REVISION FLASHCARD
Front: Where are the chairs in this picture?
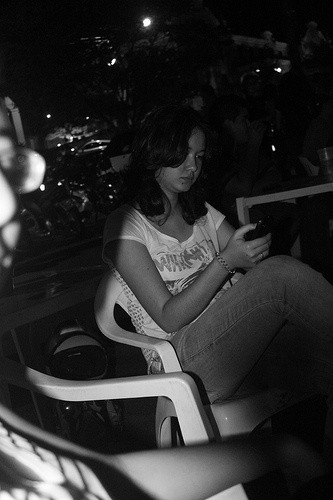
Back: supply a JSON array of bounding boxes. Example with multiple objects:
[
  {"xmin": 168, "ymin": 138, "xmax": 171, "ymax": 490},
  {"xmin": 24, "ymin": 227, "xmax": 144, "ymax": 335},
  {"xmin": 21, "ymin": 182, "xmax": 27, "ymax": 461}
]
[
  {"xmin": 1, "ymin": 358, "xmax": 248, "ymax": 500},
  {"xmin": 94, "ymin": 272, "xmax": 314, "ymax": 450}
]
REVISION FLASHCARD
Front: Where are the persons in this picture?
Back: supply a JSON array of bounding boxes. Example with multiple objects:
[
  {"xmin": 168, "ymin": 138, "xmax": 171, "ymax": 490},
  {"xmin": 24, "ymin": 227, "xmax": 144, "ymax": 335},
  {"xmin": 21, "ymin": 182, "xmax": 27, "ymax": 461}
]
[
  {"xmin": 0, "ymin": 95, "xmax": 331, "ymax": 500},
  {"xmin": 101, "ymin": 105, "xmax": 333, "ymax": 472},
  {"xmin": 75, "ymin": 1, "xmax": 333, "ymax": 284}
]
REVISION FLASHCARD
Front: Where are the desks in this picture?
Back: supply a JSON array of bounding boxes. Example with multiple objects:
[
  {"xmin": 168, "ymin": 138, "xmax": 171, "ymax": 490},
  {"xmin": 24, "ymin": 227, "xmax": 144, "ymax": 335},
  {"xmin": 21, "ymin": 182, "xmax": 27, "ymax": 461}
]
[
  {"xmin": 235, "ymin": 176, "xmax": 333, "ymax": 228},
  {"xmin": 0, "ymin": 275, "xmax": 102, "ymax": 433}
]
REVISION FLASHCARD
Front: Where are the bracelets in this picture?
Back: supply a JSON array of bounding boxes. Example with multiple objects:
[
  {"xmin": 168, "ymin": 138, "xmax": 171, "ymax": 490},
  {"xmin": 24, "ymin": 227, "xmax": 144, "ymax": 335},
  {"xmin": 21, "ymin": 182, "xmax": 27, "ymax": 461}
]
[{"xmin": 214, "ymin": 252, "xmax": 236, "ymax": 274}]
